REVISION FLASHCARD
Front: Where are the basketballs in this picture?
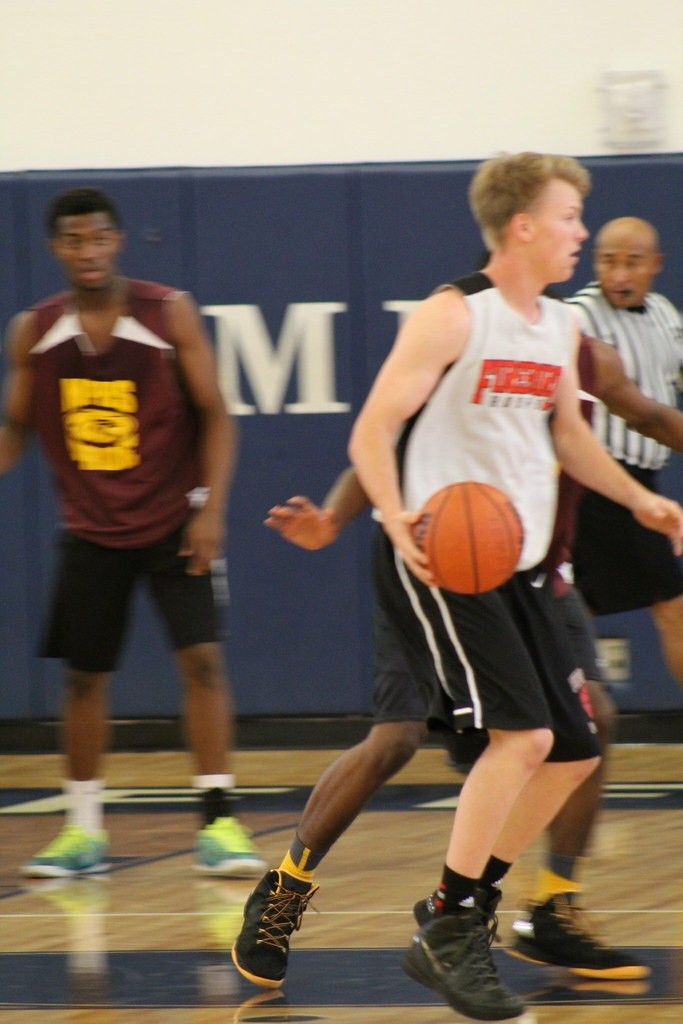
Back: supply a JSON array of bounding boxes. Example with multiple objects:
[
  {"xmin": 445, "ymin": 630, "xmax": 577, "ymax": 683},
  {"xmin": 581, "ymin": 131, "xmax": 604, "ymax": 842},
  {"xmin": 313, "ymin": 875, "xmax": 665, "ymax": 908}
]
[{"xmin": 410, "ymin": 481, "xmax": 525, "ymax": 595}]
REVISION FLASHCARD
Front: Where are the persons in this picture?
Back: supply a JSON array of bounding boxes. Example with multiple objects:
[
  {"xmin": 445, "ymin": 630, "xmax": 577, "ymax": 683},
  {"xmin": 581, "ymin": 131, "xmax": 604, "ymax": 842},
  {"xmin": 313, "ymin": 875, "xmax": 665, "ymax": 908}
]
[
  {"xmin": 232, "ymin": 250, "xmax": 683, "ymax": 989},
  {"xmin": 349, "ymin": 149, "xmax": 683, "ymax": 1021},
  {"xmin": 0, "ymin": 189, "xmax": 269, "ymax": 880},
  {"xmin": 562, "ymin": 217, "xmax": 683, "ymax": 679}
]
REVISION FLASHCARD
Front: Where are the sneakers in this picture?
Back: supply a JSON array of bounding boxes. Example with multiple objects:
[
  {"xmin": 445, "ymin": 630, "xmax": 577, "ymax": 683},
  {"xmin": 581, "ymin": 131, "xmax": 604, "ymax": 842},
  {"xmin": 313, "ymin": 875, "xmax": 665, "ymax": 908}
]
[
  {"xmin": 413, "ymin": 893, "xmax": 440, "ymax": 926},
  {"xmin": 15, "ymin": 822, "xmax": 113, "ymax": 879},
  {"xmin": 507, "ymin": 867, "xmax": 653, "ymax": 980},
  {"xmin": 399, "ymin": 909, "xmax": 526, "ymax": 1020},
  {"xmin": 190, "ymin": 816, "xmax": 269, "ymax": 881},
  {"xmin": 231, "ymin": 868, "xmax": 321, "ymax": 988}
]
